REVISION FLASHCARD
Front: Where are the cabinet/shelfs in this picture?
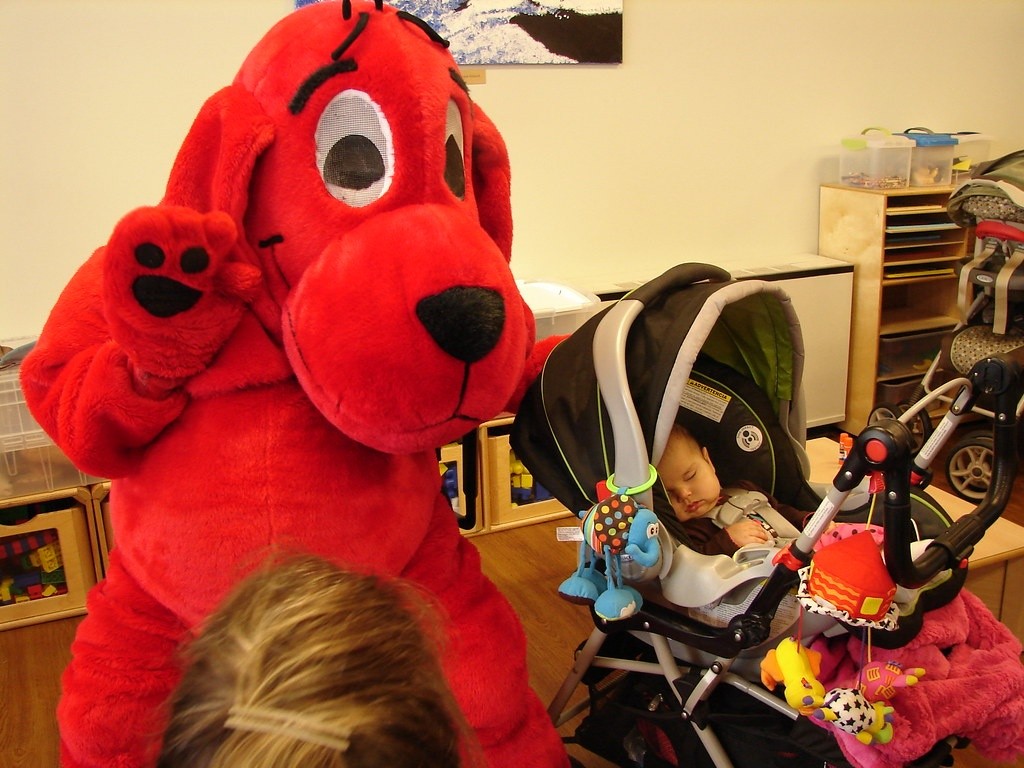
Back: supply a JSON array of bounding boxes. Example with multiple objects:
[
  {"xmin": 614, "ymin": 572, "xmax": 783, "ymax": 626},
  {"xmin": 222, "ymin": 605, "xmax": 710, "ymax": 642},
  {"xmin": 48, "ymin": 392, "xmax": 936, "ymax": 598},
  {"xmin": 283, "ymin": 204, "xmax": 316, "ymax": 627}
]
[{"xmin": 819, "ymin": 175, "xmax": 977, "ymax": 437}]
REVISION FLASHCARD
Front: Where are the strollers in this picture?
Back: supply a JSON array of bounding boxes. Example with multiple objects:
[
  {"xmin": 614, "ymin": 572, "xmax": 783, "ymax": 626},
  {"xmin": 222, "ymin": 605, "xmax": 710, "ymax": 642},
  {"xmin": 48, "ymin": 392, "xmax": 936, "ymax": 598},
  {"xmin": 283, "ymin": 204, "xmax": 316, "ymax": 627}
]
[
  {"xmin": 863, "ymin": 149, "xmax": 1024, "ymax": 505},
  {"xmin": 506, "ymin": 261, "xmax": 1024, "ymax": 768}
]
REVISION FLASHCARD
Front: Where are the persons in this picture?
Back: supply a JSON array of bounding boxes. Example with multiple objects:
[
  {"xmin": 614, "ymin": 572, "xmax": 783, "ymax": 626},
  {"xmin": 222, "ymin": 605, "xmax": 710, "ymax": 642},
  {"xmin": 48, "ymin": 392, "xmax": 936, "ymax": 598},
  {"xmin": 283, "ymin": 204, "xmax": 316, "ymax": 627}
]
[
  {"xmin": 656, "ymin": 423, "xmax": 836, "ymax": 559},
  {"xmin": 150, "ymin": 551, "xmax": 484, "ymax": 768}
]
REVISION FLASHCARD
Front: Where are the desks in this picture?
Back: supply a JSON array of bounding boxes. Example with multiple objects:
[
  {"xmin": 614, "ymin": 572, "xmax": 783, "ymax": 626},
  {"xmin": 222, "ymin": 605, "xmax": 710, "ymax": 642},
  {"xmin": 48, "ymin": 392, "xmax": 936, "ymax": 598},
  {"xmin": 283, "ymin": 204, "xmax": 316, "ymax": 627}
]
[{"xmin": 803, "ymin": 437, "xmax": 1024, "ymax": 642}]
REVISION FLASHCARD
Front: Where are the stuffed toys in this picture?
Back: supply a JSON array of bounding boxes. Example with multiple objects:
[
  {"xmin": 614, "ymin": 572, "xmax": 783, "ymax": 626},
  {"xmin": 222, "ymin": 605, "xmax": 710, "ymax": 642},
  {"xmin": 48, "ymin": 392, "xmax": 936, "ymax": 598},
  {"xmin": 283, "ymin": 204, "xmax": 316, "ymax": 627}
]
[
  {"xmin": 813, "ymin": 626, "xmax": 894, "ymax": 747},
  {"xmin": 856, "ymin": 626, "xmax": 925, "ymax": 701},
  {"xmin": 759, "ymin": 603, "xmax": 838, "ymax": 721},
  {"xmin": 558, "ymin": 486, "xmax": 661, "ymax": 622},
  {"xmin": 18, "ymin": 1, "xmax": 578, "ymax": 768}
]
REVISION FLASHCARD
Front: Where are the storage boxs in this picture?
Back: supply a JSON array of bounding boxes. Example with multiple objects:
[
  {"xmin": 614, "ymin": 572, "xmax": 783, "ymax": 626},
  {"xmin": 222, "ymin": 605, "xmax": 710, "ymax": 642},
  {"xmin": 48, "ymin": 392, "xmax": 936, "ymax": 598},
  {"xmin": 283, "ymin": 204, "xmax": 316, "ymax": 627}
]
[
  {"xmin": 0, "ymin": 360, "xmax": 111, "ymax": 500},
  {"xmin": 892, "ymin": 126, "xmax": 958, "ymax": 187},
  {"xmin": 840, "ymin": 127, "xmax": 916, "ymax": 189},
  {"xmin": 487, "ymin": 432, "xmax": 575, "ymax": 526},
  {"xmin": 951, "ymin": 133, "xmax": 993, "ymax": 186},
  {"xmin": 438, "ymin": 441, "xmax": 482, "ymax": 535},
  {"xmin": 0, "ymin": 506, "xmax": 95, "ymax": 624}
]
